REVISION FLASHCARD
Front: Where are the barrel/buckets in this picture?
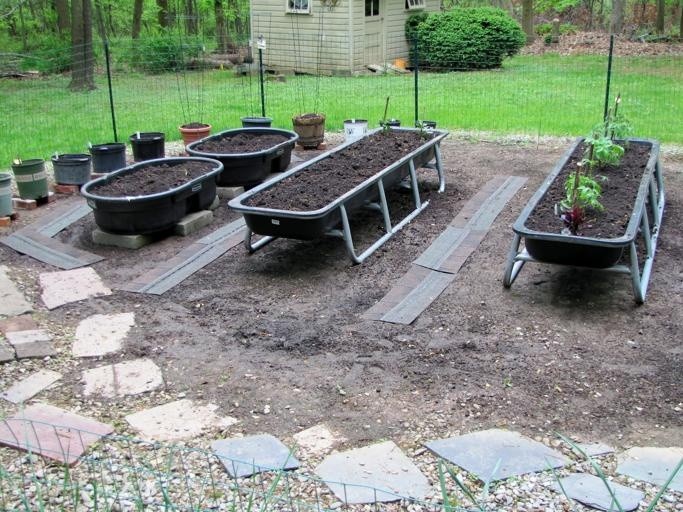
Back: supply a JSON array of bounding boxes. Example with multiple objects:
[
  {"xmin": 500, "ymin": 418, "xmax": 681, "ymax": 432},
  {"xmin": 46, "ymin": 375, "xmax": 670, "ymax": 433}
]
[
  {"xmin": 292, "ymin": 111, "xmax": 328, "ymax": 149},
  {"xmin": 0, "ymin": 174, "xmax": 12, "ymax": 218},
  {"xmin": 13, "ymin": 158, "xmax": 49, "ymax": 199},
  {"xmin": 243, "ymin": 115, "xmax": 270, "ymax": 128},
  {"xmin": 423, "ymin": 121, "xmax": 436, "ymax": 130},
  {"xmin": 129, "ymin": 128, "xmax": 167, "ymax": 159},
  {"xmin": 90, "ymin": 141, "xmax": 127, "ymax": 173},
  {"xmin": 51, "ymin": 153, "xmax": 91, "ymax": 187},
  {"xmin": 380, "ymin": 118, "xmax": 402, "ymax": 126},
  {"xmin": 343, "ymin": 119, "xmax": 367, "ymax": 141}
]
